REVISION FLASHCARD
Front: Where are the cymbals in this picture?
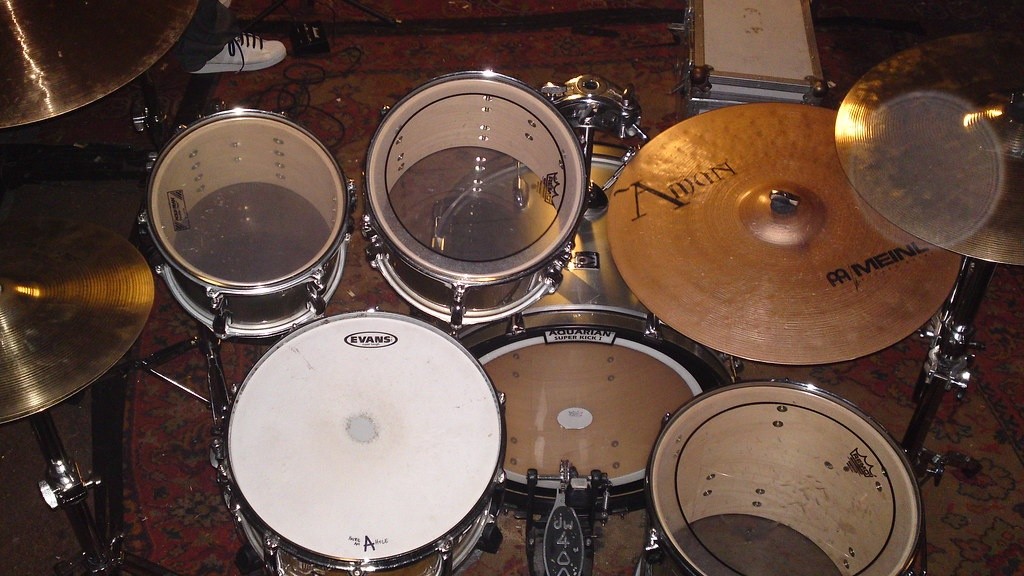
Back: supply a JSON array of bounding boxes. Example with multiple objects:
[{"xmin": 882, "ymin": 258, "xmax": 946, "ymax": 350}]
[
  {"xmin": 606, "ymin": 100, "xmax": 963, "ymax": 366},
  {"xmin": 834, "ymin": 30, "xmax": 1024, "ymax": 266},
  {"xmin": 0, "ymin": 212, "xmax": 155, "ymax": 425},
  {"xmin": 0, "ymin": 1, "xmax": 202, "ymax": 128}
]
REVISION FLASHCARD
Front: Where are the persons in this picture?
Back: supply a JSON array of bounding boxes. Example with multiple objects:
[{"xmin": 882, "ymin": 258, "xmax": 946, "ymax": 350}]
[{"xmin": 171, "ymin": 0, "xmax": 286, "ymax": 75}]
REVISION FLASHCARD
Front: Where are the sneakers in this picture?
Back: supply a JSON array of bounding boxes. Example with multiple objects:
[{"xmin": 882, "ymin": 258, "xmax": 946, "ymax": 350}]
[{"xmin": 190, "ymin": 32, "xmax": 288, "ymax": 73}]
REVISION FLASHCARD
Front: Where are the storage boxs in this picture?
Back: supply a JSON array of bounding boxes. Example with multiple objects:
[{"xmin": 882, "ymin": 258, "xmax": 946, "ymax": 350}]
[{"xmin": 666, "ymin": 0, "xmax": 830, "ymax": 123}]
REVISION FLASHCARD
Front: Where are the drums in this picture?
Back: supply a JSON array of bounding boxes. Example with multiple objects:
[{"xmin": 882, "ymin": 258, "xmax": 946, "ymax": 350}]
[
  {"xmin": 412, "ymin": 140, "xmax": 739, "ymax": 520},
  {"xmin": 217, "ymin": 308, "xmax": 507, "ymax": 576},
  {"xmin": 644, "ymin": 380, "xmax": 926, "ymax": 576},
  {"xmin": 368, "ymin": 68, "xmax": 594, "ymax": 325},
  {"xmin": 137, "ymin": 108, "xmax": 358, "ymax": 340}
]
